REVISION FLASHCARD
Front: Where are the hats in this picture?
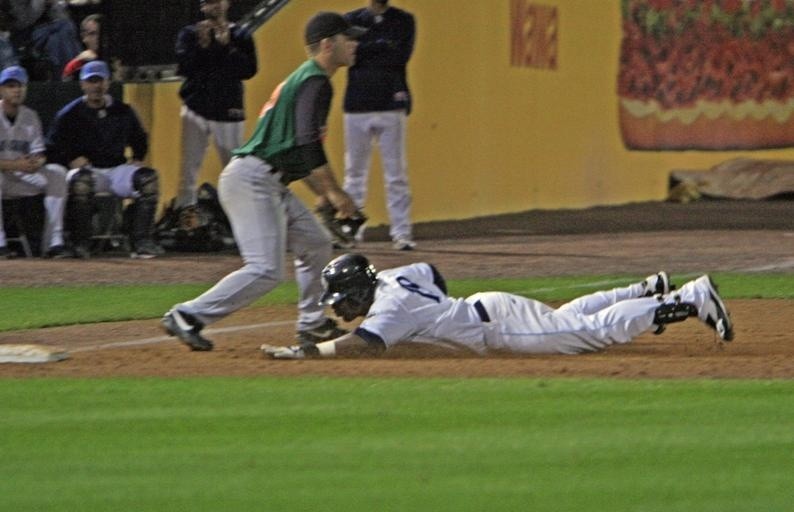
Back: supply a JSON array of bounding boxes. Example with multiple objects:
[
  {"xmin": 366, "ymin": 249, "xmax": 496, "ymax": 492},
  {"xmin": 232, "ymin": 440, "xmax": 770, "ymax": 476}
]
[
  {"xmin": 81, "ymin": 62, "xmax": 109, "ymax": 81},
  {"xmin": 0, "ymin": 65, "xmax": 28, "ymax": 84},
  {"xmin": 304, "ymin": 11, "xmax": 369, "ymax": 45}
]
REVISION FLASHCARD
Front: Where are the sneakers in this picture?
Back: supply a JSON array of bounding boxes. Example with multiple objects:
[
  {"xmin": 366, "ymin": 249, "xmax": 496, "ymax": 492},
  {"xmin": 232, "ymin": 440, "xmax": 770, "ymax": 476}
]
[
  {"xmin": 129, "ymin": 246, "xmax": 158, "ymax": 259},
  {"xmin": 393, "ymin": 234, "xmax": 416, "ymax": 251},
  {"xmin": 50, "ymin": 245, "xmax": 75, "ymax": 259},
  {"xmin": 161, "ymin": 310, "xmax": 213, "ymax": 352},
  {"xmin": 693, "ymin": 274, "xmax": 736, "ymax": 342},
  {"xmin": 295, "ymin": 318, "xmax": 349, "ymax": 341},
  {"xmin": 642, "ymin": 268, "xmax": 671, "ymax": 294}
]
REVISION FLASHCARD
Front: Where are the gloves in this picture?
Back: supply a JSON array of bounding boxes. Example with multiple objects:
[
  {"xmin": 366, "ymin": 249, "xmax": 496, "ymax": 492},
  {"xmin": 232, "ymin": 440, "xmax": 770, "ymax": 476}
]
[{"xmin": 261, "ymin": 342, "xmax": 335, "ymax": 360}]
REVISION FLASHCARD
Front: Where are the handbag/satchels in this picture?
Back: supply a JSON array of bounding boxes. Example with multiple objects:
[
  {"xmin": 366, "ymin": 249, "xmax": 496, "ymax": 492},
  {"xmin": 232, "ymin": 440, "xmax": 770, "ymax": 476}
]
[{"xmin": 174, "ymin": 204, "xmax": 224, "ymax": 252}]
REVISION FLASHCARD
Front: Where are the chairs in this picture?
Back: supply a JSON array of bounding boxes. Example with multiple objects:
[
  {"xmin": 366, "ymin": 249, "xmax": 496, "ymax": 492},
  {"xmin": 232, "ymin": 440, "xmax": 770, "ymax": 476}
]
[
  {"xmin": 47, "ymin": 189, "xmax": 139, "ymax": 257},
  {"xmin": 0, "ymin": 194, "xmax": 32, "ymax": 259}
]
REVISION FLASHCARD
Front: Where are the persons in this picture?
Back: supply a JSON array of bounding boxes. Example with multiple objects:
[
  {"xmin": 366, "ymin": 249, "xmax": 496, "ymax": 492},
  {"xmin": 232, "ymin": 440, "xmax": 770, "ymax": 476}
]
[
  {"xmin": 259, "ymin": 250, "xmax": 735, "ymax": 364},
  {"xmin": 341, "ymin": 0, "xmax": 417, "ymax": 251},
  {"xmin": 159, "ymin": 8, "xmax": 368, "ymax": 354},
  {"xmin": 1, "ymin": 1, "xmax": 257, "ymax": 258}
]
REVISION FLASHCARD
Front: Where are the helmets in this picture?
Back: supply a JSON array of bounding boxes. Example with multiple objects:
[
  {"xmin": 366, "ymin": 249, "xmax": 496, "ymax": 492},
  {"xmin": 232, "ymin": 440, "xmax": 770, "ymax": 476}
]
[{"xmin": 318, "ymin": 254, "xmax": 377, "ymax": 305}]
